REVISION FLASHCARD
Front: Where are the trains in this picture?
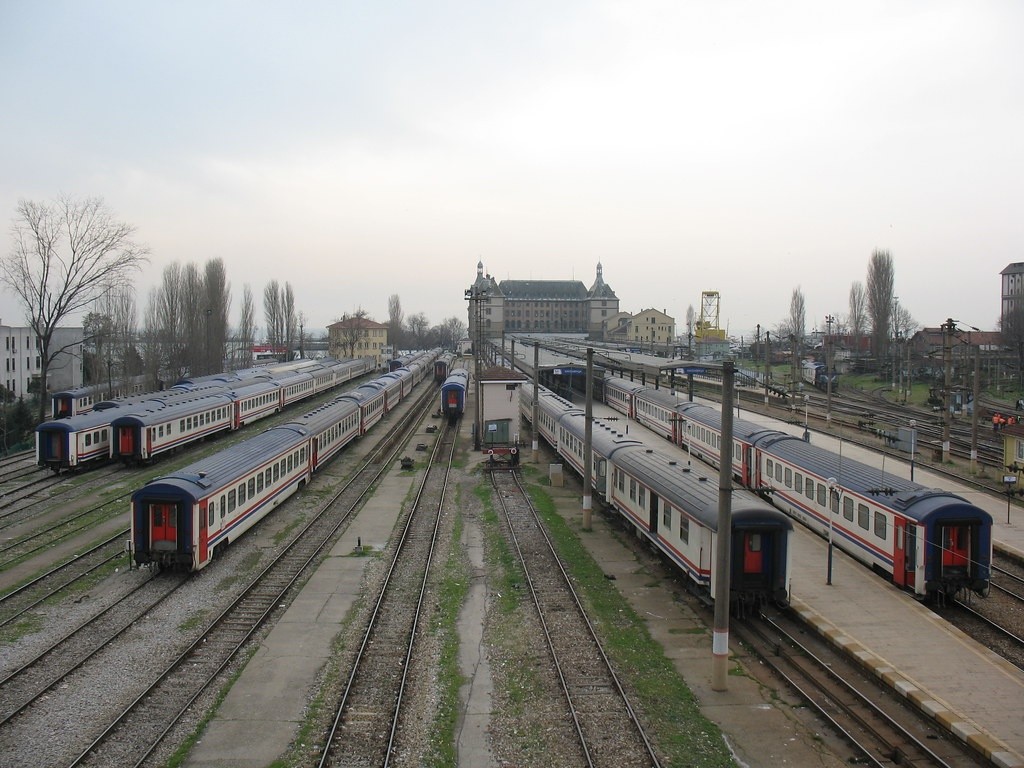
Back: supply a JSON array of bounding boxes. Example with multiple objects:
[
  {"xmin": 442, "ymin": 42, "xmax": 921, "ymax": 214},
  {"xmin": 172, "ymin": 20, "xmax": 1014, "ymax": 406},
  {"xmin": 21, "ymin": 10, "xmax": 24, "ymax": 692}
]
[
  {"xmin": 803, "ymin": 362, "xmax": 838, "ymax": 393},
  {"xmin": 34, "ymin": 347, "xmax": 470, "ymax": 576},
  {"xmin": 483, "ymin": 344, "xmax": 794, "ymax": 610},
  {"xmin": 602, "ymin": 376, "xmax": 995, "ymax": 612}
]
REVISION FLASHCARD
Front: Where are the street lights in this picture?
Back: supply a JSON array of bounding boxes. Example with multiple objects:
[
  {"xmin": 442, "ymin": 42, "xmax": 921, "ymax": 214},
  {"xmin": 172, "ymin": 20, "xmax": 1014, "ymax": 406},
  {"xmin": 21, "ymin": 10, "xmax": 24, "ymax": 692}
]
[
  {"xmin": 803, "ymin": 394, "xmax": 810, "ymax": 433},
  {"xmin": 735, "ymin": 381, "xmax": 743, "ymax": 418},
  {"xmin": 825, "ymin": 476, "xmax": 838, "ymax": 586},
  {"xmin": 823, "ymin": 314, "xmax": 835, "ymax": 428},
  {"xmin": 909, "ymin": 420, "xmax": 917, "ymax": 481}
]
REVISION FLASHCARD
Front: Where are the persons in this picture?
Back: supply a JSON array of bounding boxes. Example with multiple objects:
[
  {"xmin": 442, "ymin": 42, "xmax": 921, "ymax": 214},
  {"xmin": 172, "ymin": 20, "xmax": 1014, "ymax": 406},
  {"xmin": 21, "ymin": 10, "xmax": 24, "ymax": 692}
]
[
  {"xmin": 992, "ymin": 413, "xmax": 1006, "ymax": 432},
  {"xmin": 1008, "ymin": 416, "xmax": 1014, "ymax": 425}
]
